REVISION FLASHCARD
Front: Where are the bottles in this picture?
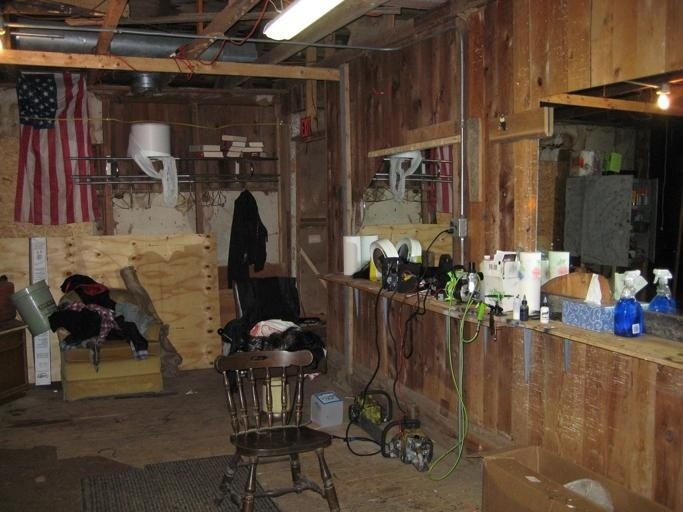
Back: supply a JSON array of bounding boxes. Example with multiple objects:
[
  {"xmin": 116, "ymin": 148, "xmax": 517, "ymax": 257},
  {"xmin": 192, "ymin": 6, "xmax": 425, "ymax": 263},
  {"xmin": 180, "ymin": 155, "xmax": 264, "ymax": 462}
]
[
  {"xmin": 520, "ymin": 294, "xmax": 528, "ymax": 321},
  {"xmin": 539, "ymin": 297, "xmax": 549, "ymax": 326},
  {"xmin": 513, "ymin": 298, "xmax": 521, "ymax": 320},
  {"xmin": 479, "ymin": 255, "xmax": 492, "ymax": 302}
]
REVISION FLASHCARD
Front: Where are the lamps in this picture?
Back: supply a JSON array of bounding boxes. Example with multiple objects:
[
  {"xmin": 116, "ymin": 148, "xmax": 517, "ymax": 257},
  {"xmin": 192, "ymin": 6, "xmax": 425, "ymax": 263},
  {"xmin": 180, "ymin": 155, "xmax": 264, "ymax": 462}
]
[
  {"xmin": 656, "ymin": 90, "xmax": 671, "ymax": 111},
  {"xmin": 263, "ymin": 0, "xmax": 343, "ymax": 41}
]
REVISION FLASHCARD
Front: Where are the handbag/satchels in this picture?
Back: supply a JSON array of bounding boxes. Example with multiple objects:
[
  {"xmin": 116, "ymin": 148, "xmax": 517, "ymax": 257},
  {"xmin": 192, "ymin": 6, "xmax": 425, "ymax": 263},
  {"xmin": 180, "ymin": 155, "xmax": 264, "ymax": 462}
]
[{"xmin": 228, "ymin": 312, "xmax": 325, "ymax": 371}]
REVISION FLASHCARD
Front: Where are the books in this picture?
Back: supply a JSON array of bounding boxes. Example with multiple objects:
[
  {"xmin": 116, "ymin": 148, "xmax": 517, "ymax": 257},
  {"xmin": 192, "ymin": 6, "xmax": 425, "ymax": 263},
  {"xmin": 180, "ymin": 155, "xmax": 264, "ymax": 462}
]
[{"xmin": 189, "ymin": 134, "xmax": 267, "ymax": 158}]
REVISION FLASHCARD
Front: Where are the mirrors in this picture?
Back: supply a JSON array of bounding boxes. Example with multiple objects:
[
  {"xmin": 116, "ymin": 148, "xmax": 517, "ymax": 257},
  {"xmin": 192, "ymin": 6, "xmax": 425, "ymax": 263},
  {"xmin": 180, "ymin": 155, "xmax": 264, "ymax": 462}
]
[
  {"xmin": 535, "ymin": 70, "xmax": 683, "ymax": 316},
  {"xmin": 373, "ymin": 143, "xmax": 452, "ymax": 226}
]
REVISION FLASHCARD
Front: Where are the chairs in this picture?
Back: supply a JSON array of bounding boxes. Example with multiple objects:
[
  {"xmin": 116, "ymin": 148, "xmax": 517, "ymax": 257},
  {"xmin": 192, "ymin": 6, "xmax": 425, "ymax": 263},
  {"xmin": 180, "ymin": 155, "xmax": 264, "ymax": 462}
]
[
  {"xmin": 57, "ymin": 288, "xmax": 163, "ymax": 402},
  {"xmin": 232, "ymin": 263, "xmax": 327, "ymax": 384},
  {"xmin": 214, "ymin": 350, "xmax": 341, "ymax": 512}
]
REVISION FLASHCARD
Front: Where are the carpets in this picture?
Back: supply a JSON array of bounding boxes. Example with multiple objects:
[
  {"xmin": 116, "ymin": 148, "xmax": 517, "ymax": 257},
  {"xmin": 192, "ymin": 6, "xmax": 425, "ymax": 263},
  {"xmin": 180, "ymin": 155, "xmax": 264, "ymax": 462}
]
[{"xmin": 78, "ymin": 450, "xmax": 282, "ymax": 511}]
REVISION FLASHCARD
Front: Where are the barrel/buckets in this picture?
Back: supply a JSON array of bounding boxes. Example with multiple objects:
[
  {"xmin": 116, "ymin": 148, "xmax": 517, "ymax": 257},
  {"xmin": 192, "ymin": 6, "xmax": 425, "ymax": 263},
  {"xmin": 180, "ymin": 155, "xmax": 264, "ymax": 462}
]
[{"xmin": 8, "ymin": 279, "xmax": 58, "ymax": 337}]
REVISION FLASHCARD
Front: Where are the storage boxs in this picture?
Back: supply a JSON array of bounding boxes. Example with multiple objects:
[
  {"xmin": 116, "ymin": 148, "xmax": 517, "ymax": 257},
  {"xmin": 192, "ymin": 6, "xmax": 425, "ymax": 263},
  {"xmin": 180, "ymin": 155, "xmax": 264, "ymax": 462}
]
[{"xmin": 481, "ymin": 446, "xmax": 671, "ymax": 512}]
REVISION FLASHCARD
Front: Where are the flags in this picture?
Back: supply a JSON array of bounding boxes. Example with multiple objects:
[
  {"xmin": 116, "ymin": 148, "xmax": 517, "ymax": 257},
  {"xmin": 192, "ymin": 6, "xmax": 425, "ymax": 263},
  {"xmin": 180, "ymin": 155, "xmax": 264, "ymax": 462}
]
[{"xmin": 15, "ymin": 71, "xmax": 100, "ymax": 223}]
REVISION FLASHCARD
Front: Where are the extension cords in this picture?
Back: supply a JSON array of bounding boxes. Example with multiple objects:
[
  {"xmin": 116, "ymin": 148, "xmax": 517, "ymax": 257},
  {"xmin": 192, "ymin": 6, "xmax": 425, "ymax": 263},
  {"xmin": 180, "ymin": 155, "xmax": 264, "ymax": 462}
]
[{"xmin": 465, "ymin": 308, "xmax": 511, "ymax": 323}]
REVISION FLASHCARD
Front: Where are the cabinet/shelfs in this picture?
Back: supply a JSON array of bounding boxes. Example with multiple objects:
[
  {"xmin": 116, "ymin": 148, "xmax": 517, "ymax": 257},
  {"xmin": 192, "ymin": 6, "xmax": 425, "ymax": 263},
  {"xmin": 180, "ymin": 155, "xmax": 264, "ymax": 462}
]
[
  {"xmin": 629, "ymin": 205, "xmax": 650, "ymax": 262},
  {"xmin": 68, "ymin": 156, "xmax": 194, "ymax": 209},
  {"xmin": 0, "ymin": 319, "xmax": 31, "ymax": 400}
]
